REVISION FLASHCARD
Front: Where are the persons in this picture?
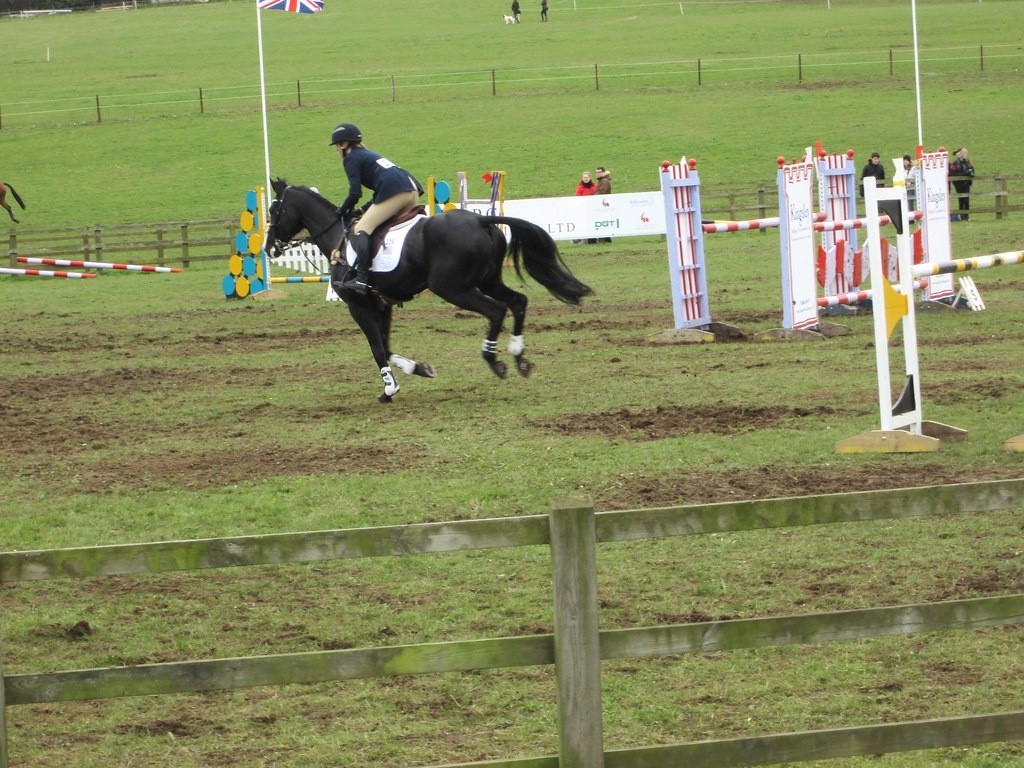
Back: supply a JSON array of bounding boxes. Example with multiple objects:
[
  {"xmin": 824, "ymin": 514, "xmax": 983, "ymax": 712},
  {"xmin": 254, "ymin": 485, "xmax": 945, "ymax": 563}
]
[
  {"xmin": 329, "ymin": 123, "xmax": 424, "ymax": 295},
  {"xmin": 540, "ymin": 0, "xmax": 548, "ymax": 22},
  {"xmin": 859, "ymin": 153, "xmax": 885, "ymax": 197},
  {"xmin": 938, "ymin": 146, "xmax": 975, "ymax": 220},
  {"xmin": 588, "ymin": 167, "xmax": 612, "ymax": 244},
  {"xmin": 512, "ymin": 0, "xmax": 519, "ymax": 17},
  {"xmin": 571, "ymin": 171, "xmax": 597, "ymax": 244},
  {"xmin": 903, "ymin": 155, "xmax": 914, "ymax": 224}
]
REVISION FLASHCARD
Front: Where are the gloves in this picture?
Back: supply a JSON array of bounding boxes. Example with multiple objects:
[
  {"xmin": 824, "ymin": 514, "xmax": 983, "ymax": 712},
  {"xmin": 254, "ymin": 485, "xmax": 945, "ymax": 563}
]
[
  {"xmin": 349, "ymin": 208, "xmax": 365, "ymax": 220},
  {"xmin": 334, "ymin": 205, "xmax": 352, "ymax": 221},
  {"xmin": 860, "ymin": 185, "xmax": 864, "ymax": 197}
]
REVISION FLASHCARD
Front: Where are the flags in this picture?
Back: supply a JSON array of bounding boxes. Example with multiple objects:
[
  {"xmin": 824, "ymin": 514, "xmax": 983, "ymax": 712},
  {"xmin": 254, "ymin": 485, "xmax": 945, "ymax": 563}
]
[{"xmin": 258, "ymin": 0, "xmax": 324, "ymax": 14}]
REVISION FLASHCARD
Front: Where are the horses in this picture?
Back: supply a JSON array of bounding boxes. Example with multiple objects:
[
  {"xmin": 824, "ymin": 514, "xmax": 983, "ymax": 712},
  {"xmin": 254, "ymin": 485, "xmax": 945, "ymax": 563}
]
[{"xmin": 263, "ymin": 176, "xmax": 596, "ymax": 405}]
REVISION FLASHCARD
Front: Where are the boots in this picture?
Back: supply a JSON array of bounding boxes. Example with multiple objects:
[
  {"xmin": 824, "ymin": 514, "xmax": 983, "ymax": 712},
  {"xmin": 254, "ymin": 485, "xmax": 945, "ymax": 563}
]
[{"xmin": 331, "ymin": 230, "xmax": 373, "ymax": 296}]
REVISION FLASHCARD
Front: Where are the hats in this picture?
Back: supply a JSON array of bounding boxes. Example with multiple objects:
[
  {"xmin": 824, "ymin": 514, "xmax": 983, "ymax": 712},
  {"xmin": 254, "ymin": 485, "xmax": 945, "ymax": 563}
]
[{"xmin": 903, "ymin": 155, "xmax": 911, "ymax": 160}]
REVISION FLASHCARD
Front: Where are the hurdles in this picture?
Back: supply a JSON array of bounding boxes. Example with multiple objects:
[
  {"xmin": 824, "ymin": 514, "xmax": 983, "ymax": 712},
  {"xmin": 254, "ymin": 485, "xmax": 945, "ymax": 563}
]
[
  {"xmin": 221, "ymin": 170, "xmax": 460, "ymax": 300},
  {"xmin": 831, "ymin": 173, "xmax": 1023, "ymax": 457},
  {"xmin": 642, "ymin": 138, "xmax": 970, "ymax": 345}
]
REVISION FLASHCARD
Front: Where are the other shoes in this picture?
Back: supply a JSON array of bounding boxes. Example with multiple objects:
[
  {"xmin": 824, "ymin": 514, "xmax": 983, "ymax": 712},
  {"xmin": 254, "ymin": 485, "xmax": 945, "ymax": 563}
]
[
  {"xmin": 588, "ymin": 239, "xmax": 597, "ymax": 244},
  {"xmin": 604, "ymin": 238, "xmax": 611, "ymax": 242}
]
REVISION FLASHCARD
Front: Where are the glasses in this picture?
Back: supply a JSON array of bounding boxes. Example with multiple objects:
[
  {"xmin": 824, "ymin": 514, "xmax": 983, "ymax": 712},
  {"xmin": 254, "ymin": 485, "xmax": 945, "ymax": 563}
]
[{"xmin": 595, "ymin": 172, "xmax": 600, "ymax": 173}]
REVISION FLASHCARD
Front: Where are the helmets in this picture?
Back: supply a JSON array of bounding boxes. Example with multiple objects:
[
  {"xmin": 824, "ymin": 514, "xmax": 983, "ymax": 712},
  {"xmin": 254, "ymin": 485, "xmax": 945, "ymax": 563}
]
[{"xmin": 329, "ymin": 123, "xmax": 362, "ymax": 146}]
